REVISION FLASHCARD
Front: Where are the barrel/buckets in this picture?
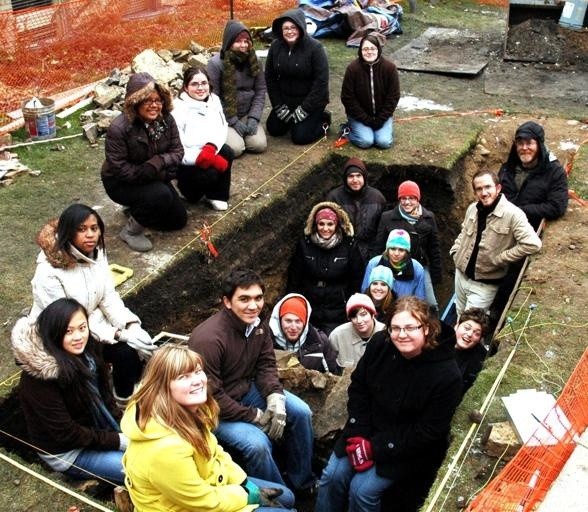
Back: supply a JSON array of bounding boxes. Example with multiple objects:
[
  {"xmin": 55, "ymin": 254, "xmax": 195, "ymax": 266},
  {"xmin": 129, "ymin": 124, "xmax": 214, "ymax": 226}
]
[{"xmin": 22, "ymin": 99, "xmax": 56, "ymax": 138}]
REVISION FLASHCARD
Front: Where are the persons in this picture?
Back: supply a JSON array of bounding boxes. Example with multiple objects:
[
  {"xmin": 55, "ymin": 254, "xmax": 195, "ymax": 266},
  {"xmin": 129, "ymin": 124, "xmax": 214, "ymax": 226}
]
[
  {"xmin": 206, "ymin": 20, "xmax": 267, "ymax": 158},
  {"xmin": 340, "ymin": 34, "xmax": 400, "ymax": 149},
  {"xmin": 167, "ymin": 65, "xmax": 234, "ymax": 212},
  {"xmin": 30, "ymin": 205, "xmax": 158, "ymax": 412},
  {"xmin": 12, "ymin": 298, "xmax": 126, "ymax": 479},
  {"xmin": 189, "ymin": 158, "xmax": 490, "ymax": 512},
  {"xmin": 265, "ymin": 9, "xmax": 332, "ymax": 146},
  {"xmin": 101, "ymin": 72, "xmax": 182, "ymax": 253},
  {"xmin": 120, "ymin": 343, "xmax": 295, "ymax": 512},
  {"xmin": 451, "ymin": 169, "xmax": 542, "ymax": 349},
  {"xmin": 490, "ymin": 122, "xmax": 568, "ymax": 333}
]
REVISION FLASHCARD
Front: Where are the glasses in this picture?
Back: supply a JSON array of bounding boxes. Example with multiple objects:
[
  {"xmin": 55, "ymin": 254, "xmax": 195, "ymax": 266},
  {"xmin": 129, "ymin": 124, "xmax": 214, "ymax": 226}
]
[
  {"xmin": 142, "ymin": 98, "xmax": 165, "ymax": 105},
  {"xmin": 388, "ymin": 323, "xmax": 422, "ymax": 334},
  {"xmin": 282, "ymin": 25, "xmax": 297, "ymax": 32}
]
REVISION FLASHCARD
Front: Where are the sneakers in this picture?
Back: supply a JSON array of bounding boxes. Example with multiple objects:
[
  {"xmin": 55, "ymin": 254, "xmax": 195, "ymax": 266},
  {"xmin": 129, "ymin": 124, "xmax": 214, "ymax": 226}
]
[{"xmin": 203, "ymin": 195, "xmax": 228, "ymax": 211}]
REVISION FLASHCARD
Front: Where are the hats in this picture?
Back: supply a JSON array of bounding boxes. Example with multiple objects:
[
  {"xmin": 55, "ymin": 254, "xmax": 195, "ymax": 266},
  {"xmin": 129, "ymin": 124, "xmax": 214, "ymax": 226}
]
[
  {"xmin": 235, "ymin": 31, "xmax": 250, "ymax": 42},
  {"xmin": 398, "ymin": 180, "xmax": 421, "ymax": 202},
  {"xmin": 514, "ymin": 132, "xmax": 536, "ymax": 141},
  {"xmin": 345, "ymin": 292, "xmax": 376, "ymax": 320},
  {"xmin": 280, "ymin": 296, "xmax": 306, "ymax": 326},
  {"xmin": 316, "ymin": 207, "xmax": 338, "ymax": 226},
  {"xmin": 385, "ymin": 229, "xmax": 411, "ymax": 253},
  {"xmin": 368, "ymin": 265, "xmax": 394, "ymax": 290}
]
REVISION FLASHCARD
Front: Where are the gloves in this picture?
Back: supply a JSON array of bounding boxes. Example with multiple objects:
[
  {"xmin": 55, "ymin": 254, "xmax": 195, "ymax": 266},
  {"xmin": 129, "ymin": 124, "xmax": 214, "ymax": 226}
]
[
  {"xmin": 118, "ymin": 329, "xmax": 158, "ymax": 357},
  {"xmin": 371, "ymin": 118, "xmax": 385, "ymax": 130},
  {"xmin": 134, "ymin": 163, "xmax": 157, "ymax": 184},
  {"xmin": 166, "ymin": 166, "xmax": 179, "ymax": 179},
  {"xmin": 196, "ymin": 143, "xmax": 217, "ymax": 169},
  {"xmin": 128, "ymin": 322, "xmax": 152, "ymax": 345},
  {"xmin": 251, "ymin": 393, "xmax": 375, "ymax": 506},
  {"xmin": 232, "ymin": 104, "xmax": 311, "ymax": 137},
  {"xmin": 363, "ymin": 116, "xmax": 374, "ymax": 126},
  {"xmin": 210, "ymin": 154, "xmax": 228, "ymax": 173}
]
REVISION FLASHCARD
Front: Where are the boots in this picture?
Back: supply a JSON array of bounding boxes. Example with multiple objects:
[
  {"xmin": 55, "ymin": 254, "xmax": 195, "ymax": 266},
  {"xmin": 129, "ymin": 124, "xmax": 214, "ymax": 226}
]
[{"xmin": 119, "ymin": 214, "xmax": 153, "ymax": 252}]
